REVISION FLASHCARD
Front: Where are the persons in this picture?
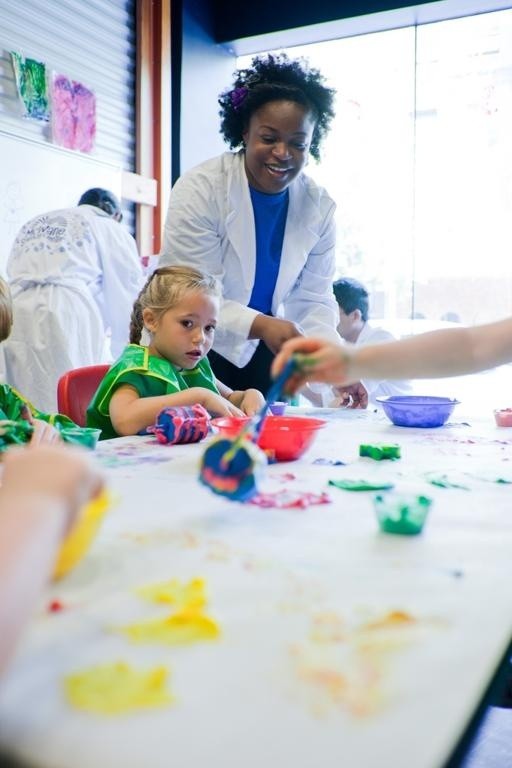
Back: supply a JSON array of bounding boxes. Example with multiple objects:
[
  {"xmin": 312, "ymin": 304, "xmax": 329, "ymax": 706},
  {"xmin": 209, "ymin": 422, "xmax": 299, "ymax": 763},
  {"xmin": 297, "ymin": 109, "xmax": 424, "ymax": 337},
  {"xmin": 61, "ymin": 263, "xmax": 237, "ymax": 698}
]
[
  {"xmin": 0, "ymin": 276, "xmax": 101, "ymax": 450},
  {"xmin": 158, "ymin": 50, "xmax": 371, "ymax": 410},
  {"xmin": 295, "ymin": 275, "xmax": 412, "ymax": 407},
  {"xmin": 269, "ymin": 312, "xmax": 512, "ymax": 387},
  {"xmin": 84, "ymin": 264, "xmax": 271, "ymax": 441},
  {"xmin": 0, "ymin": 440, "xmax": 105, "ymax": 682},
  {"xmin": 1, "ymin": 188, "xmax": 148, "ymax": 415}
]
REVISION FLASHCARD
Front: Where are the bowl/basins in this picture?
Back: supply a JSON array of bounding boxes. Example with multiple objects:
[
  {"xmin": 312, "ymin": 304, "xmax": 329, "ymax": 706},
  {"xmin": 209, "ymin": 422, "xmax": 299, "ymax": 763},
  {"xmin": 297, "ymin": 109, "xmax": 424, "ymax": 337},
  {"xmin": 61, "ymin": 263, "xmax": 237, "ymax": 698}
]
[
  {"xmin": 377, "ymin": 394, "xmax": 459, "ymax": 427},
  {"xmin": 210, "ymin": 417, "xmax": 327, "ymax": 461}
]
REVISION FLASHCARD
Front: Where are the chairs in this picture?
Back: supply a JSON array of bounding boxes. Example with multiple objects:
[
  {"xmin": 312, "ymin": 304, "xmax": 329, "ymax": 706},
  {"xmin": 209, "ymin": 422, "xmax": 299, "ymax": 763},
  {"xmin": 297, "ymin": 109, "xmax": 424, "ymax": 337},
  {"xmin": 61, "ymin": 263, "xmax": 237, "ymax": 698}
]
[{"xmin": 56, "ymin": 364, "xmax": 113, "ymax": 428}]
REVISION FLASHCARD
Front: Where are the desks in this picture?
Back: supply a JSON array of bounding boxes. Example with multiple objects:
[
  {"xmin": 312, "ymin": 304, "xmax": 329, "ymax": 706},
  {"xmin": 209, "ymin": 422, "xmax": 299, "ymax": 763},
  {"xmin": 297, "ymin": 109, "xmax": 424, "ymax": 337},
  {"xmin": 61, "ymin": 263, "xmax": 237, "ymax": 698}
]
[{"xmin": 0, "ymin": 402, "xmax": 512, "ymax": 768}]
